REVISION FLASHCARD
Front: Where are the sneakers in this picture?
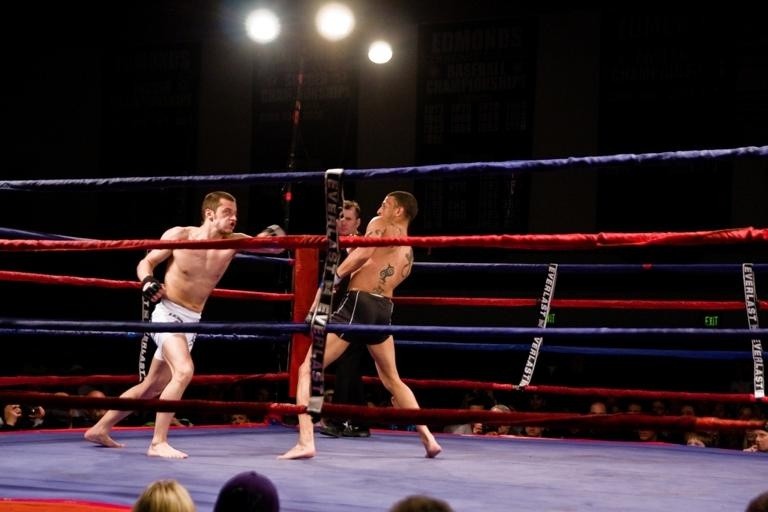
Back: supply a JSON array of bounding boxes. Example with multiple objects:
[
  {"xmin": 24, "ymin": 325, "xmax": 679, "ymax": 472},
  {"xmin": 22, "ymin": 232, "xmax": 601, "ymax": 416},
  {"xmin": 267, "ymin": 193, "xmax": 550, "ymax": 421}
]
[
  {"xmin": 319, "ymin": 424, "xmax": 340, "ymax": 437},
  {"xmin": 343, "ymin": 422, "xmax": 369, "ymax": 437}
]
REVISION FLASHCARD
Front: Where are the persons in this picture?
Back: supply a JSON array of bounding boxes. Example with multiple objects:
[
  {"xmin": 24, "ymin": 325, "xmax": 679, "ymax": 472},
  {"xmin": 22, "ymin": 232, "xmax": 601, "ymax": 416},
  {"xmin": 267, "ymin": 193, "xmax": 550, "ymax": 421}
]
[
  {"xmin": 317, "ymin": 199, "xmax": 372, "ymax": 438},
  {"xmin": 274, "ymin": 189, "xmax": 441, "ymax": 460},
  {"xmin": 132, "ymin": 476, "xmax": 198, "ymax": 512},
  {"xmin": 80, "ymin": 190, "xmax": 291, "ymax": 460},
  {"xmin": 0, "ymin": 380, "xmax": 768, "ymax": 454}
]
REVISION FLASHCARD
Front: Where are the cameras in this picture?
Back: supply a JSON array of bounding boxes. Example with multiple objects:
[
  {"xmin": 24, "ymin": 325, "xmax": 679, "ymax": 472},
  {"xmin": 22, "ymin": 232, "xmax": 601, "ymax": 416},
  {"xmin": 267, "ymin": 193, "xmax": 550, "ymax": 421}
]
[{"xmin": 21, "ymin": 406, "xmax": 38, "ymax": 416}]
